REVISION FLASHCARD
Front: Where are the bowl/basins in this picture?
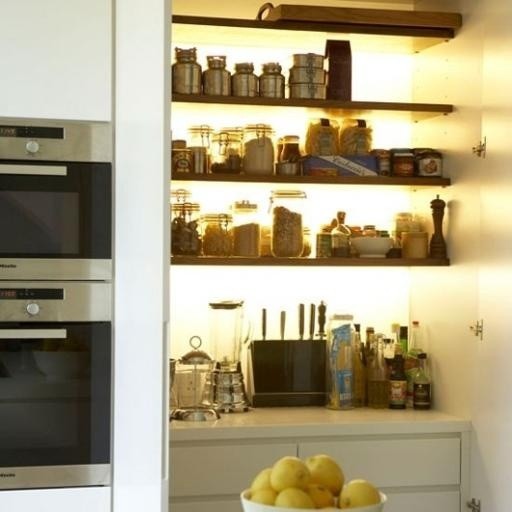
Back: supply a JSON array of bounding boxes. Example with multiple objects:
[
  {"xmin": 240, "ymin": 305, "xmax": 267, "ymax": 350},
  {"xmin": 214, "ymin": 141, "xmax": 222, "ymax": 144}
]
[
  {"xmin": 347, "ymin": 236, "xmax": 394, "ymax": 260},
  {"xmin": 238, "ymin": 484, "xmax": 391, "ymax": 510},
  {"xmin": 288, "ymin": 82, "xmax": 329, "ymax": 100},
  {"xmin": 292, "ymin": 52, "xmax": 326, "ymax": 69},
  {"xmin": 290, "ymin": 67, "xmax": 328, "ymax": 84}
]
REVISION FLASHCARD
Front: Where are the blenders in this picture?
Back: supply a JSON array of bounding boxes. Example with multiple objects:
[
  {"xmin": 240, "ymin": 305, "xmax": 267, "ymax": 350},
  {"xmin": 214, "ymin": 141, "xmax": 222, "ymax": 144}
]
[{"xmin": 202, "ymin": 300, "xmax": 251, "ymax": 416}]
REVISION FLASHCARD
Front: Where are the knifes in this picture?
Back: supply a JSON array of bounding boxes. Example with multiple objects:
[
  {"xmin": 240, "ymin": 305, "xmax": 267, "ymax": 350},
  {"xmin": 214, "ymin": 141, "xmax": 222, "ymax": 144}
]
[{"xmin": 261, "ymin": 303, "xmax": 316, "ymax": 343}]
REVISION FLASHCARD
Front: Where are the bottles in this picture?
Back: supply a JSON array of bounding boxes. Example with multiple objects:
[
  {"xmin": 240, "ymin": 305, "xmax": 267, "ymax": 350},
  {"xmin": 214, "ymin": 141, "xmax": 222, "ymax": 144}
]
[
  {"xmin": 201, "ymin": 213, "xmax": 232, "ymax": 256},
  {"xmin": 373, "ymin": 148, "xmax": 443, "ymax": 176},
  {"xmin": 400, "ymin": 230, "xmax": 429, "ymax": 261},
  {"xmin": 278, "ymin": 134, "xmax": 303, "ymax": 162},
  {"xmin": 170, "ymin": 46, "xmax": 286, "ymax": 101},
  {"xmin": 170, "ymin": 189, "xmax": 201, "ymax": 256},
  {"xmin": 188, "ymin": 125, "xmax": 215, "ymax": 146},
  {"xmin": 208, "ymin": 134, "xmax": 242, "ymax": 175},
  {"xmin": 326, "ymin": 313, "xmax": 434, "ymax": 412},
  {"xmin": 244, "ymin": 124, "xmax": 275, "ymax": 177},
  {"xmin": 230, "ymin": 202, "xmax": 261, "ymax": 259},
  {"xmin": 329, "ymin": 211, "xmax": 352, "ymax": 257},
  {"xmin": 269, "ymin": 189, "xmax": 312, "ymax": 259},
  {"xmin": 325, "ymin": 40, "xmax": 354, "ymax": 102}
]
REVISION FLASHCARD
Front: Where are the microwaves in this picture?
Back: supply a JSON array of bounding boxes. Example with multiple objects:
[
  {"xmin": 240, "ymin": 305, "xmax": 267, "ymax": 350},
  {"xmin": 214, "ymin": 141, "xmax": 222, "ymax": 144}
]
[
  {"xmin": 1, "ymin": 159, "xmax": 113, "ymax": 281},
  {"xmin": 0, "ymin": 321, "xmax": 113, "ymax": 487}
]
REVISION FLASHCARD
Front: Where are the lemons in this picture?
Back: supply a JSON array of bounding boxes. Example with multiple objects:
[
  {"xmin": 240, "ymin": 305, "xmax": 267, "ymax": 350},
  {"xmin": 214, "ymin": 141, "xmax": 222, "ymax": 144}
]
[{"xmin": 249, "ymin": 454, "xmax": 382, "ymax": 508}]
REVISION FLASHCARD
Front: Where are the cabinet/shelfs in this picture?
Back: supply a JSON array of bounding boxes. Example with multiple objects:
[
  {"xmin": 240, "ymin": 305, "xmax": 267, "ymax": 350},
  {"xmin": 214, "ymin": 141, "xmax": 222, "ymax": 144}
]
[
  {"xmin": 170, "ymin": 405, "xmax": 471, "ymax": 511},
  {"xmin": 168, "ymin": 11, "xmax": 461, "ymax": 268}
]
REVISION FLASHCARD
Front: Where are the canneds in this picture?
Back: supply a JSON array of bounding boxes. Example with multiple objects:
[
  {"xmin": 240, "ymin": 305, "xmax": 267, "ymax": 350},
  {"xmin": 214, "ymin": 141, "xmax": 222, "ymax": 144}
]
[{"xmin": 316, "ymin": 234, "xmax": 332, "ymax": 258}]
